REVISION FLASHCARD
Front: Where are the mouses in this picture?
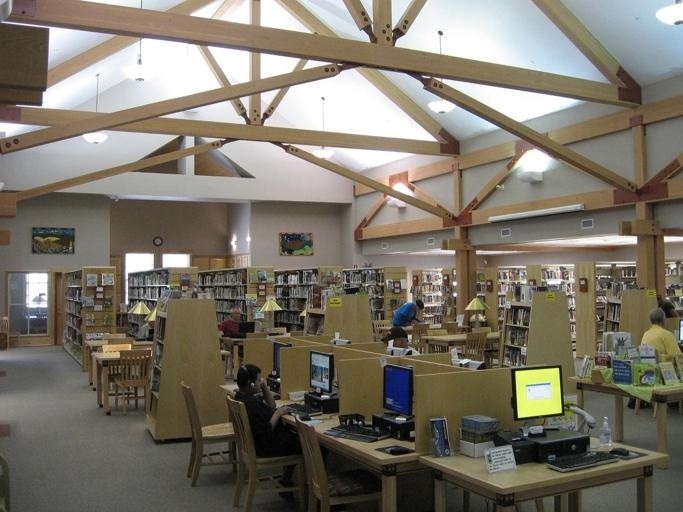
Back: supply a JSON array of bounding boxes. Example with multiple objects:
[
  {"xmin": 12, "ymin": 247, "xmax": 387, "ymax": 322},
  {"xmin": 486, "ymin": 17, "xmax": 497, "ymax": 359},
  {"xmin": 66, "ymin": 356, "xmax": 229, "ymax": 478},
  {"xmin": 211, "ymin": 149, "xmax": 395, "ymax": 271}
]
[
  {"xmin": 273, "ymin": 394, "xmax": 282, "ymax": 400},
  {"xmin": 386, "ymin": 446, "xmax": 409, "ymax": 453},
  {"xmin": 610, "ymin": 447, "xmax": 630, "ymax": 457},
  {"xmin": 300, "ymin": 415, "xmax": 312, "ymax": 421}
]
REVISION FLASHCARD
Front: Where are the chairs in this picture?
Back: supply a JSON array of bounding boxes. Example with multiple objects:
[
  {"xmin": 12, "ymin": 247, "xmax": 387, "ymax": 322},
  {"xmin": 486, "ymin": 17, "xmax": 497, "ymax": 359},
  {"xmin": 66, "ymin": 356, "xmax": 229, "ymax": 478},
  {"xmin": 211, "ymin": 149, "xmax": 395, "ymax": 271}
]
[
  {"xmin": 294, "ymin": 410, "xmax": 384, "ymax": 512},
  {"xmin": 423, "ymin": 329, "xmax": 449, "ymax": 337},
  {"xmin": 463, "ymin": 334, "xmax": 486, "ymax": 364},
  {"xmin": 370, "ymin": 318, "xmax": 391, "ymax": 342},
  {"xmin": 0, "ymin": 316, "xmax": 21, "ymax": 349},
  {"xmin": 471, "ymin": 327, "xmax": 492, "ymax": 335},
  {"xmin": 423, "ymin": 342, "xmax": 452, "ymax": 356},
  {"xmin": 178, "ymin": 381, "xmax": 239, "ymax": 487},
  {"xmin": 409, "ymin": 322, "xmax": 427, "ymax": 355},
  {"xmin": 106, "ymin": 345, "xmax": 150, "ymax": 416},
  {"xmin": 227, "ymin": 392, "xmax": 309, "ymax": 512}
]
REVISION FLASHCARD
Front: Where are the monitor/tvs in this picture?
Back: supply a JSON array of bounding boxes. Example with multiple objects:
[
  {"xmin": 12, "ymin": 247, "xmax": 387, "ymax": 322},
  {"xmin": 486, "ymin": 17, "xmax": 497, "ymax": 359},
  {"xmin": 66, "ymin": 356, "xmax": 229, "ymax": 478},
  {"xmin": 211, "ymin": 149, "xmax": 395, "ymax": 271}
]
[
  {"xmin": 384, "ymin": 347, "xmax": 413, "ymax": 356},
  {"xmin": 449, "ymin": 358, "xmax": 486, "ymax": 370},
  {"xmin": 678, "ymin": 319, "xmax": 683, "ymax": 341},
  {"xmin": 331, "ymin": 338, "xmax": 352, "ymax": 344},
  {"xmin": 272, "ymin": 341, "xmax": 293, "ymax": 370},
  {"xmin": 511, "ymin": 364, "xmax": 565, "ymax": 422},
  {"xmin": 309, "ymin": 349, "xmax": 334, "ymax": 398},
  {"xmin": 237, "ymin": 322, "xmax": 256, "ymax": 333},
  {"xmin": 382, "ymin": 363, "xmax": 414, "ymax": 417}
]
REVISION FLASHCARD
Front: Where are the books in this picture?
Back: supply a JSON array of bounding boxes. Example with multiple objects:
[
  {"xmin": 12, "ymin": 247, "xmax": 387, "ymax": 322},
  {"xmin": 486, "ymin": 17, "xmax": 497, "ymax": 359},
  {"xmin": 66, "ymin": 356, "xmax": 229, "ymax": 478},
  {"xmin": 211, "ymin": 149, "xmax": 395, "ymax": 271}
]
[
  {"xmin": 85, "ymin": 312, "xmax": 95, "ymax": 327},
  {"xmin": 596, "ymin": 325, "xmax": 604, "ymax": 342},
  {"xmin": 104, "ymin": 296, "xmax": 111, "ymax": 307},
  {"xmin": 345, "ymin": 271, "xmax": 383, "ymax": 283},
  {"xmin": 275, "ymin": 269, "xmax": 318, "ymax": 283},
  {"xmin": 127, "ymin": 286, "xmax": 167, "ymax": 299},
  {"xmin": 215, "ymin": 314, "xmax": 245, "ymax": 325},
  {"xmin": 63, "ymin": 312, "xmax": 80, "ymax": 328},
  {"xmin": 65, "ymin": 302, "xmax": 82, "ymax": 315},
  {"xmin": 412, "ymin": 285, "xmax": 441, "ymax": 292},
  {"xmin": 266, "ymin": 296, "xmax": 275, "ymax": 309},
  {"xmin": 611, "ymin": 282, "xmax": 625, "ymax": 301},
  {"xmin": 128, "ymin": 324, "xmax": 152, "ymax": 336},
  {"xmin": 501, "ymin": 283, "xmax": 518, "ymax": 292},
  {"xmin": 665, "ymin": 282, "xmax": 682, "ymax": 296},
  {"xmin": 198, "ymin": 271, "xmax": 246, "ymax": 282},
  {"xmin": 372, "ymin": 311, "xmax": 383, "ymax": 320},
  {"xmin": 258, "ymin": 285, "xmax": 265, "ymax": 297},
  {"xmin": 568, "ymin": 310, "xmax": 577, "ymax": 322},
  {"xmin": 547, "ymin": 281, "xmax": 575, "ymax": 296},
  {"xmin": 634, "ymin": 364, "xmax": 656, "ymax": 388},
  {"xmin": 607, "ymin": 303, "xmax": 621, "ymax": 320},
  {"xmin": 359, "ymin": 284, "xmax": 384, "ymax": 297},
  {"xmin": 639, "ymin": 344, "xmax": 656, "ymax": 366},
  {"xmin": 62, "ymin": 325, "xmax": 81, "ymax": 344},
  {"xmin": 413, "ymin": 294, "xmax": 441, "ymax": 304},
  {"xmin": 505, "ymin": 327, "xmax": 530, "ymax": 346},
  {"xmin": 579, "ymin": 355, "xmax": 595, "ymax": 379},
  {"xmin": 275, "ymin": 312, "xmax": 304, "ymax": 323},
  {"xmin": 596, "ymin": 280, "xmax": 609, "ymax": 291},
  {"xmin": 541, "ymin": 267, "xmax": 575, "ymax": 280},
  {"xmin": 621, "ymin": 266, "xmax": 635, "ymax": 278},
  {"xmin": 127, "ymin": 300, "xmax": 157, "ymax": 311},
  {"xmin": 86, "ymin": 274, "xmax": 96, "ymax": 287},
  {"xmin": 591, "ymin": 365, "xmax": 614, "ymax": 386},
  {"xmin": 104, "ymin": 314, "xmax": 113, "ymax": 326},
  {"xmin": 276, "ymin": 286, "xmax": 316, "ymax": 298},
  {"xmin": 94, "ymin": 305, "xmax": 103, "ymax": 312},
  {"xmin": 120, "ymin": 304, "xmax": 127, "ymax": 312},
  {"xmin": 275, "ymin": 325, "xmax": 302, "ymax": 333},
  {"xmin": 504, "ymin": 348, "xmax": 528, "ymax": 368},
  {"xmin": 86, "ymin": 296, "xmax": 94, "ymax": 306},
  {"xmin": 245, "ymin": 294, "xmax": 257, "ymax": 305},
  {"xmin": 498, "ymin": 308, "xmax": 503, "ymax": 318},
  {"xmin": 199, "ymin": 286, "xmax": 244, "ymax": 298},
  {"xmin": 386, "ymin": 278, "xmax": 407, "ymax": 294},
  {"xmin": 664, "ymin": 261, "xmax": 681, "ymax": 274},
  {"xmin": 570, "ymin": 323, "xmax": 576, "ymax": 338},
  {"xmin": 500, "ymin": 271, "xmax": 526, "ymax": 281},
  {"xmin": 607, "ymin": 321, "xmax": 619, "ymax": 331},
  {"xmin": 507, "ymin": 307, "xmax": 531, "ymax": 326},
  {"xmin": 65, "ymin": 287, "xmax": 81, "ymax": 300},
  {"xmin": 101, "ymin": 273, "xmax": 113, "ymax": 286},
  {"xmin": 612, "ymin": 354, "xmax": 632, "ymax": 383},
  {"xmin": 477, "ymin": 272, "xmax": 485, "ymax": 281},
  {"xmin": 128, "ymin": 313, "xmax": 150, "ymax": 321},
  {"xmin": 476, "ymin": 283, "xmax": 481, "ymax": 292},
  {"xmin": 128, "ymin": 270, "xmax": 168, "ymax": 285},
  {"xmin": 96, "ymin": 288, "xmax": 103, "ymax": 299},
  {"xmin": 499, "ymin": 295, "xmax": 505, "ymax": 304},
  {"xmin": 568, "ymin": 298, "xmax": 575, "ymax": 309},
  {"xmin": 592, "ymin": 352, "xmax": 614, "ymax": 367},
  {"xmin": 517, "ymin": 285, "xmax": 548, "ymax": 303},
  {"xmin": 420, "ymin": 306, "xmax": 445, "ymax": 314},
  {"xmin": 215, "ymin": 301, "xmax": 245, "ymax": 310},
  {"xmin": 412, "ymin": 272, "xmax": 443, "ymax": 284},
  {"xmin": 64, "ymin": 273, "xmax": 81, "ymax": 287},
  {"xmin": 602, "ymin": 332, "xmax": 634, "ymax": 360},
  {"xmin": 275, "ymin": 299, "xmax": 307, "ymax": 310},
  {"xmin": 369, "ymin": 299, "xmax": 383, "ymax": 309},
  {"xmin": 594, "ymin": 311, "xmax": 604, "ymax": 322},
  {"xmin": 595, "ymin": 295, "xmax": 607, "ymax": 306},
  {"xmin": 658, "ymin": 363, "xmax": 679, "ymax": 387},
  {"xmin": 249, "ymin": 271, "xmax": 274, "ymax": 284},
  {"xmin": 253, "ymin": 306, "xmax": 264, "ymax": 320}
]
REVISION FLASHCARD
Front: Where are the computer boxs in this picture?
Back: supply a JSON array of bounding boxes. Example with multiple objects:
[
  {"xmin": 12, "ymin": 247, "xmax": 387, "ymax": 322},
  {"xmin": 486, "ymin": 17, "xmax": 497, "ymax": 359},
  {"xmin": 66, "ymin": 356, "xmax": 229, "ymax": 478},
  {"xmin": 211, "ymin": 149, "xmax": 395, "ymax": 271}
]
[
  {"xmin": 303, "ymin": 393, "xmax": 339, "ymax": 413},
  {"xmin": 522, "ymin": 427, "xmax": 591, "ymax": 462},
  {"xmin": 372, "ymin": 414, "xmax": 415, "ymax": 441},
  {"xmin": 267, "ymin": 378, "xmax": 280, "ymax": 395}
]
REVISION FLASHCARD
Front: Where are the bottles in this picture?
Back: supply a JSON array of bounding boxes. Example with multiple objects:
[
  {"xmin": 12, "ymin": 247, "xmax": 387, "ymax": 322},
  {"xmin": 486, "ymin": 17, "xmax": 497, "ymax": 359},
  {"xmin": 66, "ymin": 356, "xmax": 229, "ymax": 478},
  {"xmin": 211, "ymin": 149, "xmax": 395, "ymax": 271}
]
[{"xmin": 600, "ymin": 417, "xmax": 612, "ymax": 450}]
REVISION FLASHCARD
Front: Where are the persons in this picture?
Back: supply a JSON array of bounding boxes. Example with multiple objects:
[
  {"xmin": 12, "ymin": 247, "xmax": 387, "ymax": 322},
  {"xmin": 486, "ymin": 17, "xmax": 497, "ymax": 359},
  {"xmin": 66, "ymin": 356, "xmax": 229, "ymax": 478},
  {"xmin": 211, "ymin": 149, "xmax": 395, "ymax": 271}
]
[
  {"xmin": 656, "ymin": 296, "xmax": 679, "ymax": 317},
  {"xmin": 222, "ymin": 310, "xmax": 244, "ymax": 375},
  {"xmin": 639, "ymin": 310, "xmax": 681, "ymax": 360},
  {"xmin": 392, "ymin": 300, "xmax": 425, "ymax": 341},
  {"xmin": 234, "ymin": 364, "xmax": 330, "ymax": 499},
  {"xmin": 381, "ymin": 327, "xmax": 423, "ymax": 358}
]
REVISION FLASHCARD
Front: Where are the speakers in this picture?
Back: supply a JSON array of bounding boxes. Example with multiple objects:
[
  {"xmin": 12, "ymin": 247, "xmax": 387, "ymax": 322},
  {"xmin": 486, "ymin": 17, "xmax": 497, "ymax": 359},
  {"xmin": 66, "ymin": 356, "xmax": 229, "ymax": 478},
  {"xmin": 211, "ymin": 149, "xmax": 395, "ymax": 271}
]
[{"xmin": 493, "ymin": 432, "xmax": 536, "ymax": 464}]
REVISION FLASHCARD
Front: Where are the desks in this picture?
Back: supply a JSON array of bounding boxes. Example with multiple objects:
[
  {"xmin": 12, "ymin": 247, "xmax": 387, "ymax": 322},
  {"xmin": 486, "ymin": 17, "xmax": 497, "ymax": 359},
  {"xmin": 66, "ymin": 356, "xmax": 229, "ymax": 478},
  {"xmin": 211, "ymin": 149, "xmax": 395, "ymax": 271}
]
[
  {"xmin": 220, "ymin": 372, "xmax": 670, "ymax": 512},
  {"xmin": 424, "ymin": 331, "xmax": 468, "ymax": 345},
  {"xmin": 89, "ymin": 339, "xmax": 155, "ymax": 407},
  {"xmin": 569, "ymin": 369, "xmax": 683, "ymax": 468},
  {"xmin": 419, "ymin": 428, "xmax": 670, "ymax": 512}
]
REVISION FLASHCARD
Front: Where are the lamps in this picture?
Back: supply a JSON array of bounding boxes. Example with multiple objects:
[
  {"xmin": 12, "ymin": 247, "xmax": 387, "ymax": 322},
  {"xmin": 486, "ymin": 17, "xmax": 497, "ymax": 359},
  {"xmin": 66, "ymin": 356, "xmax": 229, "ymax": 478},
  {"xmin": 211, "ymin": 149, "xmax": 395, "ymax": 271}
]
[
  {"xmin": 655, "ymin": 2, "xmax": 683, "ymax": 32},
  {"xmin": 313, "ymin": 94, "xmax": 337, "ymax": 161},
  {"xmin": 425, "ymin": 26, "xmax": 457, "ymax": 114},
  {"xmin": 128, "ymin": 1, "xmax": 153, "ymax": 82},
  {"xmin": 514, "ymin": 147, "xmax": 554, "ymax": 178},
  {"xmin": 385, "ymin": 182, "xmax": 415, "ymax": 211},
  {"xmin": 83, "ymin": 73, "xmax": 110, "ymax": 143}
]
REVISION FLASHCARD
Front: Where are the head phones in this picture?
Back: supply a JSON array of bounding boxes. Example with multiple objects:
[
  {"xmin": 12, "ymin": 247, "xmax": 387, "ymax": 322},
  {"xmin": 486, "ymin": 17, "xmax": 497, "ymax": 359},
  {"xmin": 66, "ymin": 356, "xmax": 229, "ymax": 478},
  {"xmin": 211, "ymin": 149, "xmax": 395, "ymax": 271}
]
[{"xmin": 242, "ymin": 363, "xmax": 256, "ymax": 389}]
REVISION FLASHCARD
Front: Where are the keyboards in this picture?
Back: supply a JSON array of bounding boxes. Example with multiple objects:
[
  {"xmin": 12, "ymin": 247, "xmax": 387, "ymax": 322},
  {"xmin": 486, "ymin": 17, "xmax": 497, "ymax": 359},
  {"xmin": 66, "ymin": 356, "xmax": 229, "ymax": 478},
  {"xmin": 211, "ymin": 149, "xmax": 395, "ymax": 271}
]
[
  {"xmin": 545, "ymin": 450, "xmax": 620, "ymax": 473},
  {"xmin": 331, "ymin": 421, "xmax": 391, "ymax": 440},
  {"xmin": 287, "ymin": 403, "xmax": 324, "ymax": 418}
]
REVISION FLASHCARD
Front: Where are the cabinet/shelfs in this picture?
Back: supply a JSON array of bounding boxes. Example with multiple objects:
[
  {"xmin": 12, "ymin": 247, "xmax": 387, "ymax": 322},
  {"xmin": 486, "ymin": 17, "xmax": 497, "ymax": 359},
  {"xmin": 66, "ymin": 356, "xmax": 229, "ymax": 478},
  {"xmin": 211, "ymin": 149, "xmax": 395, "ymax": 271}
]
[
  {"xmin": 665, "ymin": 263, "xmax": 683, "ymax": 317},
  {"xmin": 497, "ymin": 263, "xmax": 543, "ymax": 332},
  {"xmin": 474, "ymin": 267, "xmax": 499, "ymax": 332},
  {"xmin": 60, "ymin": 265, "xmax": 117, "ymax": 367},
  {"xmin": 603, "ymin": 287, "xmax": 660, "ymax": 356},
  {"xmin": 306, "ymin": 287, "xmax": 374, "ymax": 346},
  {"xmin": 574, "ymin": 263, "xmax": 637, "ymax": 358},
  {"xmin": 498, "ymin": 293, "xmax": 576, "ymax": 396},
  {"xmin": 199, "ymin": 265, "xmax": 275, "ymax": 333},
  {"xmin": 126, "ymin": 266, "xmax": 199, "ymax": 338},
  {"xmin": 143, "ymin": 294, "xmax": 232, "ymax": 443},
  {"xmin": 273, "ymin": 267, "xmax": 342, "ymax": 334},
  {"xmin": 344, "ymin": 267, "xmax": 405, "ymax": 323},
  {"xmin": 542, "ymin": 262, "xmax": 576, "ymax": 351},
  {"xmin": 412, "ymin": 268, "xmax": 459, "ymax": 323}
]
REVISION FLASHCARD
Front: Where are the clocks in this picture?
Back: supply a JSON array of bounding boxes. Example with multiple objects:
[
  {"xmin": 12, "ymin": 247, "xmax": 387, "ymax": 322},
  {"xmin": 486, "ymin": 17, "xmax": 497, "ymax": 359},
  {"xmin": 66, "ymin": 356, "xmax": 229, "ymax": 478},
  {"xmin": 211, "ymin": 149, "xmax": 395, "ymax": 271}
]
[{"xmin": 153, "ymin": 236, "xmax": 163, "ymax": 248}]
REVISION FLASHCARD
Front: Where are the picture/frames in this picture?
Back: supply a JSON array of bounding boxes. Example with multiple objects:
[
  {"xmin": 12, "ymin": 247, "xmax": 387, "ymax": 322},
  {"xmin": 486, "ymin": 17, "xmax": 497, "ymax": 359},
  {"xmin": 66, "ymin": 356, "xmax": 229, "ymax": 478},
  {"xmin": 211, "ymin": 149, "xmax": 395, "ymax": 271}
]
[
  {"xmin": 30, "ymin": 227, "xmax": 74, "ymax": 254},
  {"xmin": 277, "ymin": 231, "xmax": 313, "ymax": 254}
]
